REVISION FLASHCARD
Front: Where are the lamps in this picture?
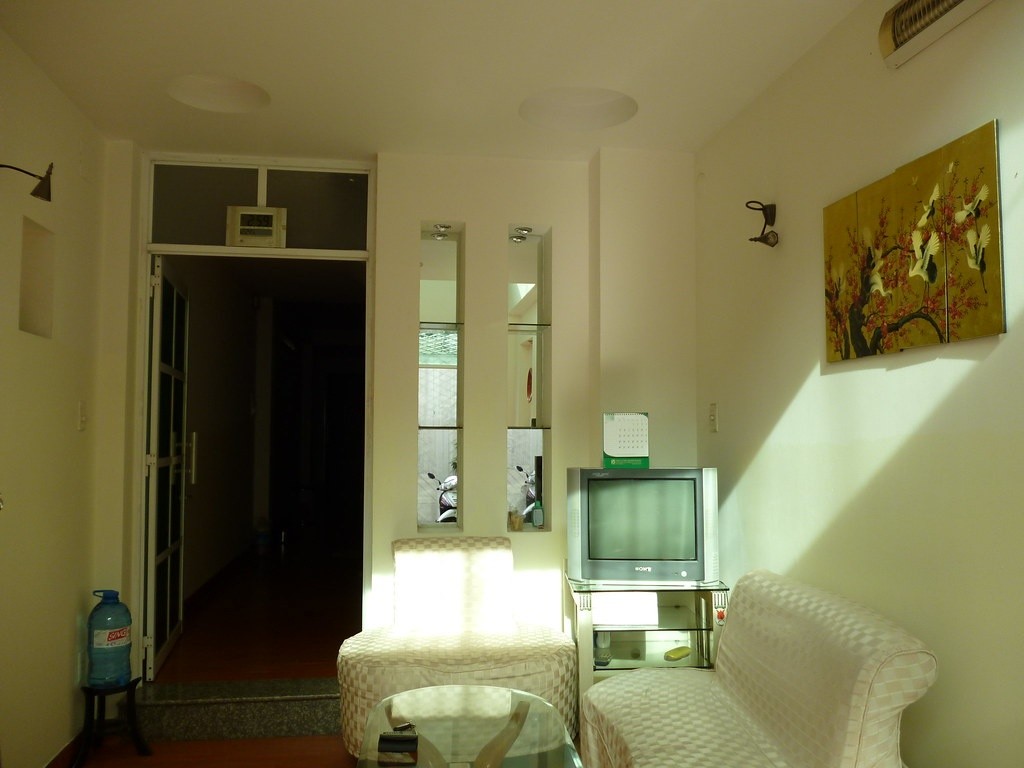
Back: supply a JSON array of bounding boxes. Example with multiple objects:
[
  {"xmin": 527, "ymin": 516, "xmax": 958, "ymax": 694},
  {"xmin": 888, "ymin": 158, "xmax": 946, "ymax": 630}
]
[
  {"xmin": 746, "ymin": 201, "xmax": 778, "ymax": 247},
  {"xmin": 0, "ymin": 162, "xmax": 54, "ymax": 203}
]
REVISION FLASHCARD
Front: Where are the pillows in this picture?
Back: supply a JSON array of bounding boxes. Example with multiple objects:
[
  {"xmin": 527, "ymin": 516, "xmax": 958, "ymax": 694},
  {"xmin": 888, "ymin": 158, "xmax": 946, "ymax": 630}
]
[{"xmin": 390, "ymin": 536, "xmax": 514, "ymax": 639}]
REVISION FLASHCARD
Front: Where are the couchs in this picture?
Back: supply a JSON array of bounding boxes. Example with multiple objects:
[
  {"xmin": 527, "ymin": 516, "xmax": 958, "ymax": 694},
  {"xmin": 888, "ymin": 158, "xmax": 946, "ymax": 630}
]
[{"xmin": 578, "ymin": 567, "xmax": 937, "ymax": 768}]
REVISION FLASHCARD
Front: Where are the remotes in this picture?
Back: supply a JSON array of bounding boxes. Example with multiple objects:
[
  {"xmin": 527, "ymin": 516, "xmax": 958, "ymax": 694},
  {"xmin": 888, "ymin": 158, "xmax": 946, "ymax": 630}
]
[{"xmin": 595, "ymin": 656, "xmax": 612, "ymax": 666}]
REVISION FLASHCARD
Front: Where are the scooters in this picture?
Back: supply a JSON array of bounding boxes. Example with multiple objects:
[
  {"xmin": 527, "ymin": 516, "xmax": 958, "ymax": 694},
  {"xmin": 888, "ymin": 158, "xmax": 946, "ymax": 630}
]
[
  {"xmin": 516, "ymin": 465, "xmax": 537, "ymax": 525},
  {"xmin": 427, "ymin": 463, "xmax": 458, "ymax": 524}
]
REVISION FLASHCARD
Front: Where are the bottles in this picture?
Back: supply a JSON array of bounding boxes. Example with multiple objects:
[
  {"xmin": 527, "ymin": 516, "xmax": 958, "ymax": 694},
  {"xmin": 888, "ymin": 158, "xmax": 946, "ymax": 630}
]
[
  {"xmin": 87, "ymin": 590, "xmax": 132, "ymax": 691},
  {"xmin": 511, "ymin": 507, "xmax": 524, "ymax": 531},
  {"xmin": 532, "ymin": 501, "xmax": 544, "ymax": 527}
]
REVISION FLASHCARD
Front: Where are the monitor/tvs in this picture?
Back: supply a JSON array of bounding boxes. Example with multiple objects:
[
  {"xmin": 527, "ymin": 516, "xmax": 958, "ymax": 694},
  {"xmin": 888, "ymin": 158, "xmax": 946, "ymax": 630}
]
[{"xmin": 567, "ymin": 467, "xmax": 720, "ymax": 588}]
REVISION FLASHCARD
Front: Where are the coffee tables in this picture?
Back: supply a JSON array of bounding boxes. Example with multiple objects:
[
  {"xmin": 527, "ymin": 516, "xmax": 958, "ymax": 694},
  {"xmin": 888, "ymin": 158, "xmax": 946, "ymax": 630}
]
[{"xmin": 359, "ymin": 683, "xmax": 584, "ymax": 767}]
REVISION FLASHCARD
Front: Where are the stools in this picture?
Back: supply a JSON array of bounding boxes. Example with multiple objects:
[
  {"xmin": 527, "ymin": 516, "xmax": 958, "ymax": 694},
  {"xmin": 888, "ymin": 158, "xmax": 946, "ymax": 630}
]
[{"xmin": 339, "ymin": 631, "xmax": 579, "ymax": 757}]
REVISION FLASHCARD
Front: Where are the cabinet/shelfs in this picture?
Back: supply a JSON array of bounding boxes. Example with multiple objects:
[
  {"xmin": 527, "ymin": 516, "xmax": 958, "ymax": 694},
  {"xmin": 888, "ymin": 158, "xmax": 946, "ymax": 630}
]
[
  {"xmin": 507, "ymin": 322, "xmax": 547, "ymax": 426},
  {"xmin": 564, "ymin": 561, "xmax": 731, "ymax": 761},
  {"xmin": 419, "ymin": 321, "xmax": 461, "ymax": 430}
]
